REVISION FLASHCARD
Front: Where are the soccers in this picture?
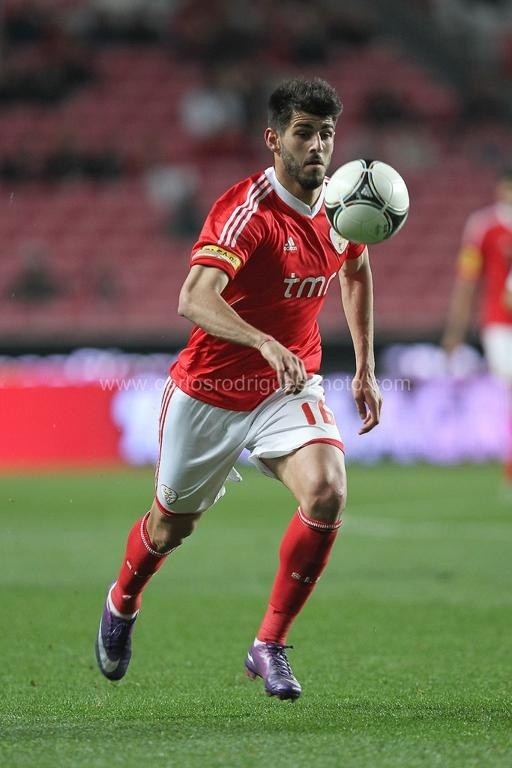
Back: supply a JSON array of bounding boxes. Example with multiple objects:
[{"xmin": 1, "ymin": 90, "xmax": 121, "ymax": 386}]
[{"xmin": 323, "ymin": 158, "xmax": 410, "ymax": 246}]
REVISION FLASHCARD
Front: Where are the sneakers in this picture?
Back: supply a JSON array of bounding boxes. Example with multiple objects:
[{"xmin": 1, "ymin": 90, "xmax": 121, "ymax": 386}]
[
  {"xmin": 246, "ymin": 636, "xmax": 301, "ymax": 703},
  {"xmin": 95, "ymin": 582, "xmax": 140, "ymax": 680}
]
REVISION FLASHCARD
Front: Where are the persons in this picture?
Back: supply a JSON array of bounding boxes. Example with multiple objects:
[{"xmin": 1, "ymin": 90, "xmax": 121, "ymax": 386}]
[
  {"xmin": 0, "ymin": 0, "xmax": 512, "ymax": 310},
  {"xmin": 434, "ymin": 158, "xmax": 511, "ymax": 477},
  {"xmin": 92, "ymin": 73, "xmax": 384, "ymax": 701}
]
